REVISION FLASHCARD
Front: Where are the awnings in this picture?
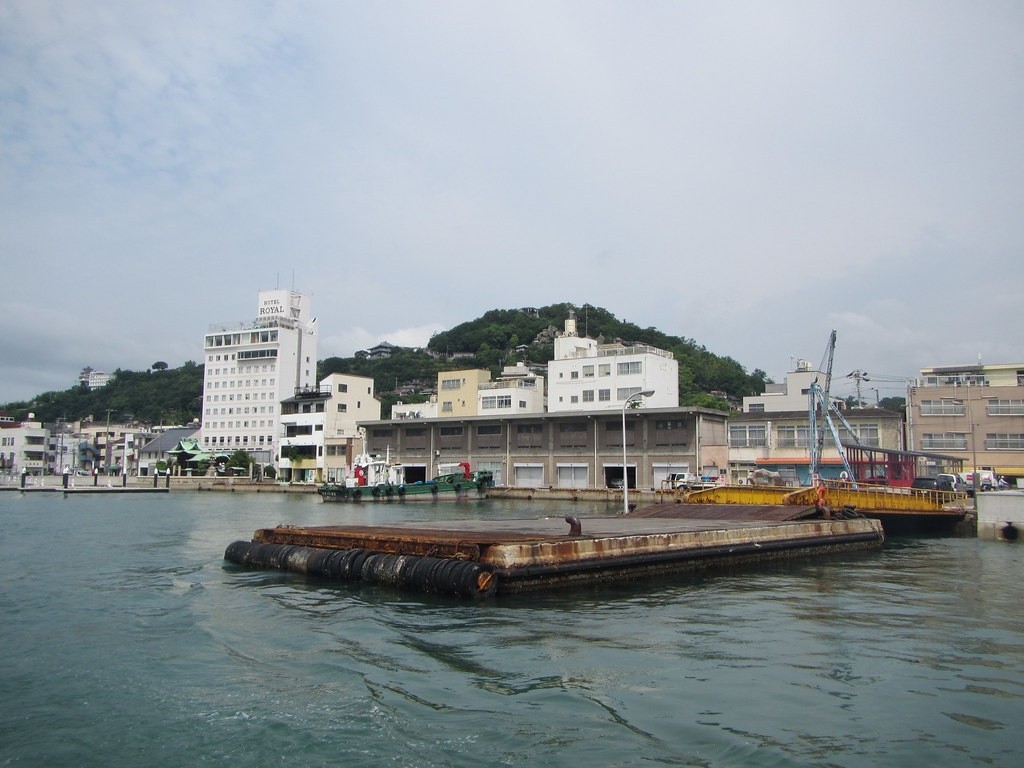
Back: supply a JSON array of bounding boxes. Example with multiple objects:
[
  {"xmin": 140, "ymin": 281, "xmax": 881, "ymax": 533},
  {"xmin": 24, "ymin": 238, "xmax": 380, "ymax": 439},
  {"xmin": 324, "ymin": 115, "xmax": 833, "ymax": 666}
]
[{"xmin": 109, "ymin": 465, "xmax": 120, "ymax": 470}]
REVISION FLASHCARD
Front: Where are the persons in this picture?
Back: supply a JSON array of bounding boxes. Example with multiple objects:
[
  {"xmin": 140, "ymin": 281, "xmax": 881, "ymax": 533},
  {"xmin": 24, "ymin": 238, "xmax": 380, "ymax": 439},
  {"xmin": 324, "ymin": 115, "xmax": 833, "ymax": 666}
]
[
  {"xmin": 384, "ymin": 470, "xmax": 389, "ymax": 484},
  {"xmin": 999, "ymin": 475, "xmax": 1008, "ymax": 488}
]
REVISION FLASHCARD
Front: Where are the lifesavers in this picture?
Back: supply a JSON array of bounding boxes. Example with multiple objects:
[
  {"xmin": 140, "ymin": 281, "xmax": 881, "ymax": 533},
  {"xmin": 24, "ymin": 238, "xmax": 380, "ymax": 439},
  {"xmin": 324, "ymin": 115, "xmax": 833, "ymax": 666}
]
[
  {"xmin": 386, "ymin": 487, "xmax": 394, "ymax": 496},
  {"xmin": 359, "ymin": 470, "xmax": 364, "ymax": 477},
  {"xmin": 1003, "ymin": 526, "xmax": 1018, "ymax": 540},
  {"xmin": 431, "ymin": 485, "xmax": 438, "ymax": 493},
  {"xmin": 486, "ymin": 475, "xmax": 491, "ymax": 482},
  {"xmin": 454, "ymin": 484, "xmax": 461, "ymax": 491},
  {"xmin": 477, "ymin": 481, "xmax": 483, "ymax": 489},
  {"xmin": 341, "ymin": 486, "xmax": 348, "ymax": 494},
  {"xmin": 353, "ymin": 489, "xmax": 362, "ymax": 498},
  {"xmin": 224, "ymin": 541, "xmax": 498, "ymax": 606},
  {"xmin": 398, "ymin": 487, "xmax": 406, "ymax": 496},
  {"xmin": 372, "ymin": 487, "xmax": 380, "ymax": 496},
  {"xmin": 843, "ymin": 509, "xmax": 859, "ymax": 519},
  {"xmin": 840, "ymin": 471, "xmax": 849, "ymax": 481}
]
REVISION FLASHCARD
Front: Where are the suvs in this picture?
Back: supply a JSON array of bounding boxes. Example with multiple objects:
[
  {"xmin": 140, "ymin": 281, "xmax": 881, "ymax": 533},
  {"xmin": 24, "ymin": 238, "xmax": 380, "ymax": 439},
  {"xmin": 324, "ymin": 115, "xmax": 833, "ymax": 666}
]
[{"xmin": 912, "ymin": 476, "xmax": 956, "ymax": 502}]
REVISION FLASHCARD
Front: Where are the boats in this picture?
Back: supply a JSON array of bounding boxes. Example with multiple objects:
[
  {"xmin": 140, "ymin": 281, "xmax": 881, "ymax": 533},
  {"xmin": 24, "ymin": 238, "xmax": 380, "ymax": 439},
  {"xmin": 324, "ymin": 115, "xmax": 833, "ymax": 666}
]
[{"xmin": 317, "ymin": 469, "xmax": 493, "ymax": 502}]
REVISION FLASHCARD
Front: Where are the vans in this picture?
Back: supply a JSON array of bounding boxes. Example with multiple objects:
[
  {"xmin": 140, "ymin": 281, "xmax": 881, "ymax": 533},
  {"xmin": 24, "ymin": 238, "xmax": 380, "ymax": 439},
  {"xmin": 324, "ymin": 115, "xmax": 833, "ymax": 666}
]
[{"xmin": 937, "ymin": 473, "xmax": 968, "ymax": 494}]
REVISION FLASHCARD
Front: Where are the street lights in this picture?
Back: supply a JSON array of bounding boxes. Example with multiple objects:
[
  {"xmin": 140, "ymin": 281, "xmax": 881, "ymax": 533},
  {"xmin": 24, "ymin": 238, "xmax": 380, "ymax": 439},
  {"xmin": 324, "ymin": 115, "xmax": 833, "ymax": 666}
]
[{"xmin": 623, "ymin": 389, "xmax": 656, "ymax": 514}]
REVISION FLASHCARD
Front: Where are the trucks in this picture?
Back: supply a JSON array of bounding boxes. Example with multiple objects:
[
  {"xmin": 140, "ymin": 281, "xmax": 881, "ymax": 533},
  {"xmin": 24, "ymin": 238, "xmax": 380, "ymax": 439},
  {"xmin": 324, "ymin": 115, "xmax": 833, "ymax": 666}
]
[
  {"xmin": 666, "ymin": 473, "xmax": 715, "ymax": 491},
  {"xmin": 961, "ymin": 469, "xmax": 999, "ymax": 498}
]
